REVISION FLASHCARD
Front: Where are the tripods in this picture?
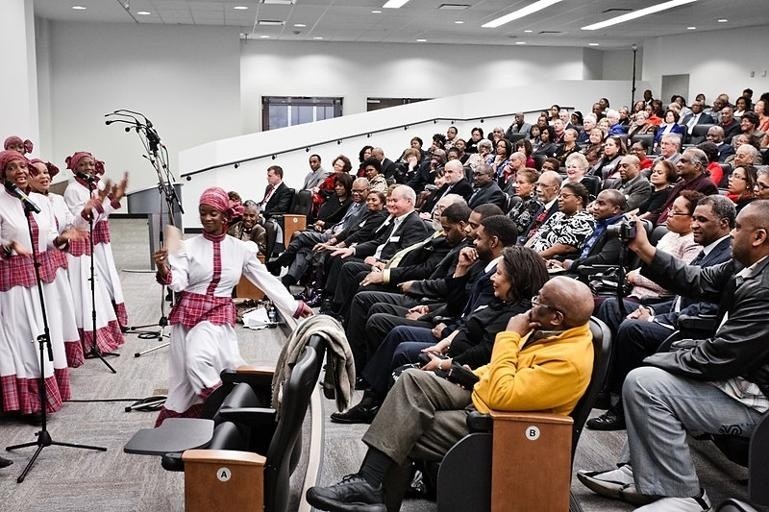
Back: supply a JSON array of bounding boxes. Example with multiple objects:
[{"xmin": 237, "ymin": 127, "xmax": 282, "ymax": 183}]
[
  {"xmin": 132, "ymin": 157, "xmax": 172, "ymax": 340},
  {"xmin": 84, "ymin": 182, "xmax": 120, "ymax": 373},
  {"xmin": 6, "ymin": 213, "xmax": 107, "ymax": 483}
]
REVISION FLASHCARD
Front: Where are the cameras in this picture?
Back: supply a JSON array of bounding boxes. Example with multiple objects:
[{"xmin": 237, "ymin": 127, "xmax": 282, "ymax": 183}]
[{"xmin": 607, "ymin": 220, "xmax": 636, "ymax": 240}]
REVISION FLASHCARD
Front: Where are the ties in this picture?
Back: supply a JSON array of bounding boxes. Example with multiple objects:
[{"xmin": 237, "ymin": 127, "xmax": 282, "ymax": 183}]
[{"xmin": 261, "ymin": 188, "xmax": 273, "ymax": 206}]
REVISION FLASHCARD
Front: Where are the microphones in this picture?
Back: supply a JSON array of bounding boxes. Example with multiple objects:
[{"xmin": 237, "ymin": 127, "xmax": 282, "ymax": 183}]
[
  {"xmin": 4, "ymin": 180, "xmax": 41, "ymax": 213},
  {"xmin": 76, "ymin": 171, "xmax": 94, "ymax": 182},
  {"xmin": 104, "ymin": 108, "xmax": 160, "ymax": 144}
]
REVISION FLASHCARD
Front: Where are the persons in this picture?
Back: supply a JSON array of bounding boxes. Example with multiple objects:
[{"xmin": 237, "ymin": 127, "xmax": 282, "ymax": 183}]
[
  {"xmin": 228, "ymin": 88, "xmax": 768, "ymax": 511},
  {"xmin": 0, "ymin": 136, "xmax": 128, "ymax": 424},
  {"xmin": 151, "ymin": 187, "xmax": 314, "ymax": 427}
]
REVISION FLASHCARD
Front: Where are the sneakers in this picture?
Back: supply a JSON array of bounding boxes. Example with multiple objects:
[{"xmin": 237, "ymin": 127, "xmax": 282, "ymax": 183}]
[
  {"xmin": 577, "ymin": 462, "xmax": 650, "ymax": 504},
  {"xmin": 306, "ymin": 474, "xmax": 388, "ymax": 512},
  {"xmin": 630, "ymin": 488, "xmax": 714, "ymax": 512}
]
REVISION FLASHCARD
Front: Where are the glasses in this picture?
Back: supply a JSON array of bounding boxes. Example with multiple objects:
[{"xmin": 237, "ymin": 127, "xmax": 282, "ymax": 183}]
[
  {"xmin": 531, "ymin": 295, "xmax": 560, "ymax": 313},
  {"xmin": 667, "ymin": 207, "xmax": 689, "ymax": 216}
]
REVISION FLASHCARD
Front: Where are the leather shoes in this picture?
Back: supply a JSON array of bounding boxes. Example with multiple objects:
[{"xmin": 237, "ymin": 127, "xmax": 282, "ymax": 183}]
[
  {"xmin": 266, "ymin": 261, "xmax": 280, "ymax": 276},
  {"xmin": 587, "ymin": 407, "xmax": 625, "ymax": 429},
  {"xmin": 355, "ymin": 376, "xmax": 370, "ymax": 390},
  {"xmin": 292, "ymin": 288, "xmax": 325, "ymax": 307},
  {"xmin": 331, "ymin": 404, "xmax": 378, "ymax": 423}
]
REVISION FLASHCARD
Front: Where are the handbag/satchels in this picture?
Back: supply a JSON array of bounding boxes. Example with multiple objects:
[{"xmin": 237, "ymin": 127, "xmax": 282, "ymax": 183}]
[{"xmin": 589, "ymin": 266, "xmax": 632, "ymax": 297}]
